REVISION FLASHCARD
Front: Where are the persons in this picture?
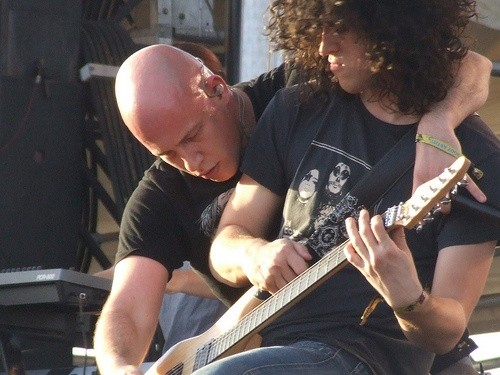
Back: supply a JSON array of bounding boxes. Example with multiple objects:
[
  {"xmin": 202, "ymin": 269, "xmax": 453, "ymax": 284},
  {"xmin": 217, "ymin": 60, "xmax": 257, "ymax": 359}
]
[
  {"xmin": 74, "ymin": 258, "xmax": 230, "ymax": 375},
  {"xmin": 93, "ymin": 42, "xmax": 252, "ymax": 355},
  {"xmin": 93, "ymin": 44, "xmax": 493, "ymax": 374},
  {"xmin": 189, "ymin": 0, "xmax": 500, "ymax": 375}
]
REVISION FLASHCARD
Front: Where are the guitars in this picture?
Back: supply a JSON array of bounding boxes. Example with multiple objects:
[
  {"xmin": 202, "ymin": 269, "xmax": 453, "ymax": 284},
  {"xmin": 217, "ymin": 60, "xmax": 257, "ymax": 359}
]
[{"xmin": 141, "ymin": 155, "xmax": 472, "ymax": 374}]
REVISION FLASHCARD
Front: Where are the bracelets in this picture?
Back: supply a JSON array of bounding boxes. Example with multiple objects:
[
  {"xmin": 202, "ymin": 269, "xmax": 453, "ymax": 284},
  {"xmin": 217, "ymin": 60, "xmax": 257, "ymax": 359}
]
[
  {"xmin": 414, "ymin": 133, "xmax": 484, "ymax": 179},
  {"xmin": 393, "ymin": 290, "xmax": 428, "ymax": 316}
]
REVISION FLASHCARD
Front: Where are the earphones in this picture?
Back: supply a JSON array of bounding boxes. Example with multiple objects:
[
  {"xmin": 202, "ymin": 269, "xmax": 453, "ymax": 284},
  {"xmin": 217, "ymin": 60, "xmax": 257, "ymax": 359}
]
[{"xmin": 210, "ymin": 83, "xmax": 224, "ymax": 97}]
